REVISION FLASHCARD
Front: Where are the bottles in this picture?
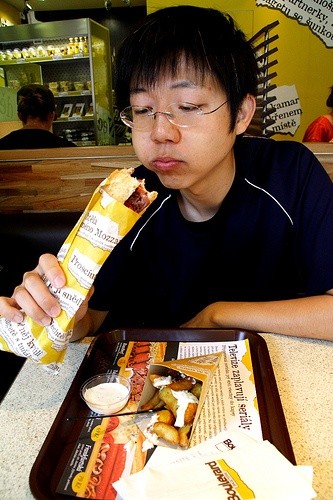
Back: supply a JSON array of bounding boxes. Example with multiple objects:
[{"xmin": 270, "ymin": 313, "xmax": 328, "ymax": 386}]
[{"xmin": 67, "ymin": 36, "xmax": 88, "ymax": 55}]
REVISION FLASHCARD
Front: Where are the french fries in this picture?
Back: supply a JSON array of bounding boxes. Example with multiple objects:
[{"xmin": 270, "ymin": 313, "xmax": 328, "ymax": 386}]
[{"xmin": 139, "ymin": 369, "xmax": 204, "ymax": 449}]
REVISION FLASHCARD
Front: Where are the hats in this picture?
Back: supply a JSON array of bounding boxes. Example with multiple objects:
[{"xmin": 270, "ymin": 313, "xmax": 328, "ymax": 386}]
[{"xmin": 17, "ymin": 85, "xmax": 55, "ymax": 110}]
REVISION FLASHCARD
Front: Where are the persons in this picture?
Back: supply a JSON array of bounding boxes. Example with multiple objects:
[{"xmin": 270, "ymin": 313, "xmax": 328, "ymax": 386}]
[
  {"xmin": 301, "ymin": 87, "xmax": 333, "ymax": 145},
  {"xmin": 0, "ymin": 4, "xmax": 333, "ymax": 342},
  {"xmin": 0, "ymin": 83, "xmax": 77, "ymax": 149}
]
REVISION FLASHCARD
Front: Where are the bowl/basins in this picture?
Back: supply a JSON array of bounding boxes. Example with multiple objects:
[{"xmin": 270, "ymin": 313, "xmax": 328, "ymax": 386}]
[{"xmin": 80, "ymin": 373, "xmax": 131, "ymax": 415}]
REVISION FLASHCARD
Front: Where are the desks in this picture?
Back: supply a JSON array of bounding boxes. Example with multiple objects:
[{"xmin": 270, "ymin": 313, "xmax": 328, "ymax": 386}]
[{"xmin": 0, "ymin": 328, "xmax": 333, "ymax": 500}]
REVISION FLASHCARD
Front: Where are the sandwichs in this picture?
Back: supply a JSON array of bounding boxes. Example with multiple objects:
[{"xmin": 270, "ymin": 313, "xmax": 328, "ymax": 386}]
[{"xmin": 101, "ymin": 167, "xmax": 158, "ymax": 216}]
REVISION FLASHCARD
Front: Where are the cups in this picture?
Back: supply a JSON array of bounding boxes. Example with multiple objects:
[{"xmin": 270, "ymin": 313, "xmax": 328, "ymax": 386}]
[{"xmin": 49, "ymin": 81, "xmax": 92, "ymax": 93}]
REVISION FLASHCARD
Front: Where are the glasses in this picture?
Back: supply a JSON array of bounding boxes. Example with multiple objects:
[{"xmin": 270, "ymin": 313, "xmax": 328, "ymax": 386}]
[{"xmin": 119, "ymin": 101, "xmax": 228, "ymax": 130}]
